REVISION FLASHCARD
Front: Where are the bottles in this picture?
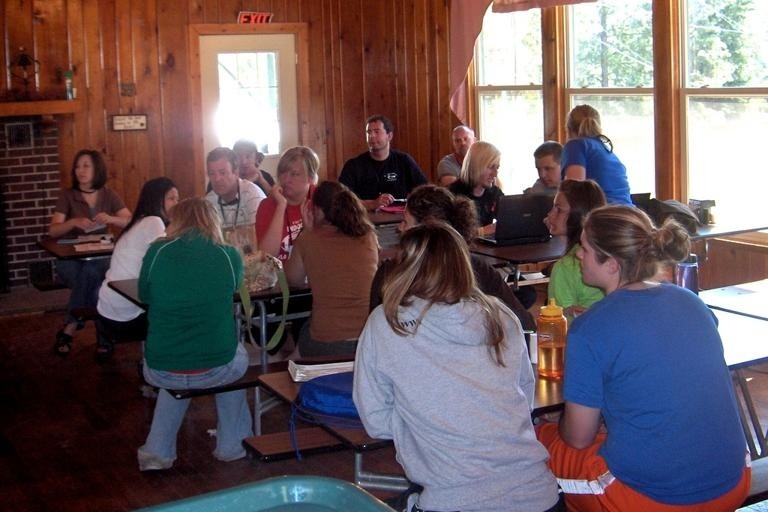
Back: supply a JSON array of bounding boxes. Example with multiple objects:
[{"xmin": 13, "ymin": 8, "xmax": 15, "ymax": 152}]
[
  {"xmin": 62, "ymin": 69, "xmax": 76, "ymax": 102},
  {"xmin": 674, "ymin": 249, "xmax": 698, "ymax": 298},
  {"xmin": 536, "ymin": 296, "xmax": 569, "ymax": 378}
]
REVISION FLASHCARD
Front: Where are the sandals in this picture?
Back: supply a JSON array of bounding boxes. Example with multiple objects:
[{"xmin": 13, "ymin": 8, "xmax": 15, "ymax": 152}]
[
  {"xmin": 54, "ymin": 328, "xmax": 74, "ymax": 357},
  {"xmin": 95, "ymin": 335, "xmax": 115, "ymax": 357}
]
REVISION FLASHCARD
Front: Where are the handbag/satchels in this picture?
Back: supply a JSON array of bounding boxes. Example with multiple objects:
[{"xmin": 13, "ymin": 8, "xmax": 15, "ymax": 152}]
[
  {"xmin": 289, "ymin": 370, "xmax": 367, "ymax": 432},
  {"xmin": 648, "ymin": 196, "xmax": 703, "ymax": 239},
  {"xmin": 236, "ymin": 249, "xmax": 285, "ymax": 293}
]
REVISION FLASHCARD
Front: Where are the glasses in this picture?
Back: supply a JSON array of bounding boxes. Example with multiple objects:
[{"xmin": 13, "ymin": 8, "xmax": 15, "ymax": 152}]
[{"xmin": 217, "ymin": 194, "xmax": 239, "ymax": 207}]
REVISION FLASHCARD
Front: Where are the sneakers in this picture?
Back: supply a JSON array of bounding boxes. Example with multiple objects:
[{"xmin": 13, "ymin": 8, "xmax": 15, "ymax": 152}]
[{"xmin": 139, "ymin": 383, "xmax": 160, "ymax": 399}]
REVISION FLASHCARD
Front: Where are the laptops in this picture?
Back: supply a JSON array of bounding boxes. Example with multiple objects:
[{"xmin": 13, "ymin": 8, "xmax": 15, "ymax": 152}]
[{"xmin": 477, "ymin": 194, "xmax": 555, "ymax": 246}]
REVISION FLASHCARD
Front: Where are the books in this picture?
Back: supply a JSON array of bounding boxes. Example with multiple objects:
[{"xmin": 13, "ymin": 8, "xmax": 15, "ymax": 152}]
[
  {"xmin": 286, "ymin": 359, "xmax": 355, "ymax": 383},
  {"xmin": 72, "ymin": 232, "xmax": 114, "ymax": 252}
]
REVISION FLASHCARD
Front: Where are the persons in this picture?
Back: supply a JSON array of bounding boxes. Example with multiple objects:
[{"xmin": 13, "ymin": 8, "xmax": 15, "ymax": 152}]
[
  {"xmin": 208, "ymin": 139, "xmax": 379, "ymax": 361},
  {"xmin": 338, "ymin": 115, "xmax": 427, "ymax": 210},
  {"xmin": 369, "ymin": 185, "xmax": 534, "ymax": 354},
  {"xmin": 135, "ymin": 194, "xmax": 255, "ymax": 473},
  {"xmin": 94, "ymin": 173, "xmax": 181, "ymax": 342},
  {"xmin": 352, "ymin": 222, "xmax": 567, "ymax": 512},
  {"xmin": 527, "ymin": 105, "xmax": 632, "ymax": 323},
  {"xmin": 47, "ymin": 147, "xmax": 134, "ymax": 361},
  {"xmin": 437, "ymin": 126, "xmax": 505, "ymax": 234},
  {"xmin": 530, "ymin": 203, "xmax": 755, "ymax": 512}
]
